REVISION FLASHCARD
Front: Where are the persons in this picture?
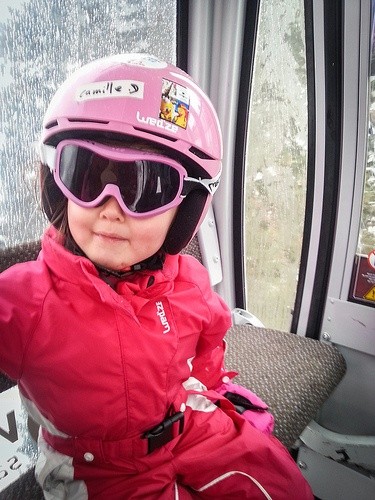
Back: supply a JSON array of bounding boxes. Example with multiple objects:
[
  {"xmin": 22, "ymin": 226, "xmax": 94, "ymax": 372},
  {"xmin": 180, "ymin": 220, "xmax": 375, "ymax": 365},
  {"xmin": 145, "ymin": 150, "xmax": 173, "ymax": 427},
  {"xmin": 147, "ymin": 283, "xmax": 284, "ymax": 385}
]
[{"xmin": 0, "ymin": 51, "xmax": 314, "ymax": 500}]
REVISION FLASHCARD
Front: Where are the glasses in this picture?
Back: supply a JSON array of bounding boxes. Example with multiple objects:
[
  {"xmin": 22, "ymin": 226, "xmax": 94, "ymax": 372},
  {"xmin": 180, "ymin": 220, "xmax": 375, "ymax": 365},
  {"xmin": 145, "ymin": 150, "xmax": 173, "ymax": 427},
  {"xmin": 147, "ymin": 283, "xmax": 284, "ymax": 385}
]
[{"xmin": 38, "ymin": 138, "xmax": 198, "ymax": 219}]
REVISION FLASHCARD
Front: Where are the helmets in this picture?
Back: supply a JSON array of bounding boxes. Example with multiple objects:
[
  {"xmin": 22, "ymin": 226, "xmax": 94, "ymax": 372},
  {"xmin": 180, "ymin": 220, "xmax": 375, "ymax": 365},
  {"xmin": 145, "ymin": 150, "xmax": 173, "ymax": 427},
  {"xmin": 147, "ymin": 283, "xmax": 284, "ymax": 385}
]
[{"xmin": 38, "ymin": 50, "xmax": 222, "ymax": 257}]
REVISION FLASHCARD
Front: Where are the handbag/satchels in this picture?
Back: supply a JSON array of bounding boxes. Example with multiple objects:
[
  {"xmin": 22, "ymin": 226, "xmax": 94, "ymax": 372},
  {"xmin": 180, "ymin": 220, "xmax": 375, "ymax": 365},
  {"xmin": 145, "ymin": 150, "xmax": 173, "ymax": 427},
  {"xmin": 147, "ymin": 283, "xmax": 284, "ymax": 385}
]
[{"xmin": 219, "ymin": 381, "xmax": 274, "ymax": 433}]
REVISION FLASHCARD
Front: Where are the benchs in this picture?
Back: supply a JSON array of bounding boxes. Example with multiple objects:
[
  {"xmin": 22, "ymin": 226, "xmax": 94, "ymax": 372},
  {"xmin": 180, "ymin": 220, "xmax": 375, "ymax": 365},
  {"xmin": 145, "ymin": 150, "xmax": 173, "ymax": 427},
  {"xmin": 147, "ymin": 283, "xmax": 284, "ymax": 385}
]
[{"xmin": 1, "ymin": 238, "xmax": 349, "ymax": 500}]
